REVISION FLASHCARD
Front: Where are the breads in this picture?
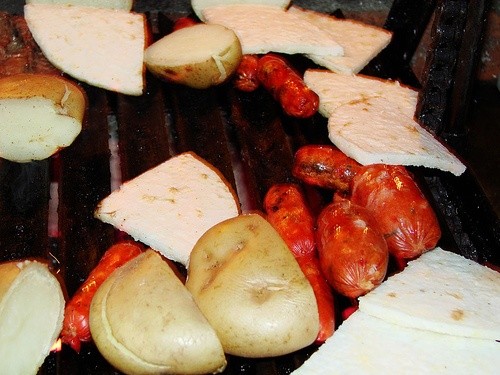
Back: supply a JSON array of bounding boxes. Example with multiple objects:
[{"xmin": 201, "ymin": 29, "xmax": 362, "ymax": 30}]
[
  {"xmin": 92, "ymin": 150, "xmax": 243, "ymax": 269},
  {"xmin": 24, "ymin": 3, "xmax": 145, "ymax": 96},
  {"xmin": 193, "ymin": 0, "xmax": 345, "ymax": 55},
  {"xmin": 286, "ymin": 5, "xmax": 393, "ymax": 74}
]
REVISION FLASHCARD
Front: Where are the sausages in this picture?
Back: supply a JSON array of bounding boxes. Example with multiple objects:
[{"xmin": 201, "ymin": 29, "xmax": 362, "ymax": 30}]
[
  {"xmin": 62, "ymin": 243, "xmax": 144, "ymax": 351},
  {"xmin": 235, "ymin": 53, "xmax": 442, "ymax": 347}
]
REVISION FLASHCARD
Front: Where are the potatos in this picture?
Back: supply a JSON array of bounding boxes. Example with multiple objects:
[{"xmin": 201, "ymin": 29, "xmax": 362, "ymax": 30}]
[
  {"xmin": 184, "ymin": 214, "xmax": 320, "ymax": 359},
  {"xmin": 143, "ymin": 23, "xmax": 242, "ymax": 88},
  {"xmin": 1, "ymin": 261, "xmax": 65, "ymax": 375},
  {"xmin": 89, "ymin": 249, "xmax": 227, "ymax": 375},
  {"xmin": 1, "ymin": 74, "xmax": 87, "ymax": 163}
]
